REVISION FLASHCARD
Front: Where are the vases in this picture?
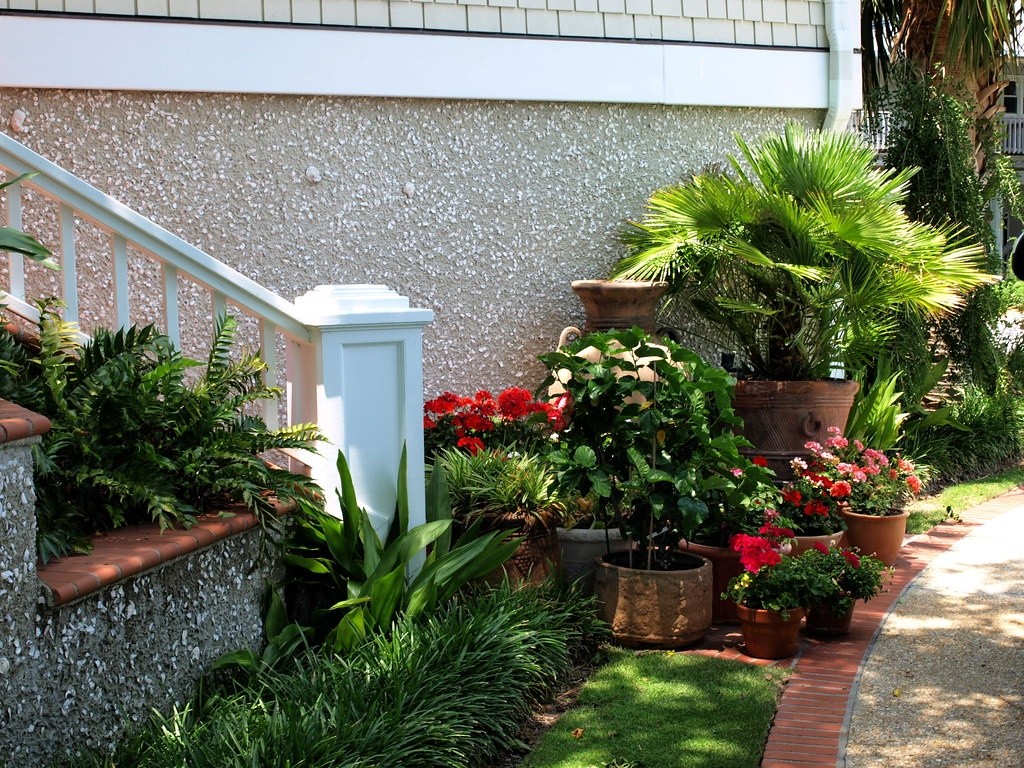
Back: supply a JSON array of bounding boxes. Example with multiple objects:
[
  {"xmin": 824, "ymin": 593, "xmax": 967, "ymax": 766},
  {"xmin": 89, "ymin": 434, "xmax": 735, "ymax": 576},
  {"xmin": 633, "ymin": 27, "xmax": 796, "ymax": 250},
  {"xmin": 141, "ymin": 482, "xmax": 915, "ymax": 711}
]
[
  {"xmin": 548, "ymin": 280, "xmax": 688, "ymax": 425},
  {"xmin": 781, "ymin": 530, "xmax": 846, "ymax": 558},
  {"xmin": 593, "ymin": 548, "xmax": 714, "ymax": 650},
  {"xmin": 843, "ymin": 508, "xmax": 912, "ymax": 566},
  {"xmin": 555, "ymin": 527, "xmax": 633, "ymax": 597},
  {"xmin": 803, "ymin": 599, "xmax": 856, "ymax": 635},
  {"xmin": 735, "ymin": 604, "xmax": 806, "ymax": 660},
  {"xmin": 678, "ymin": 539, "xmax": 793, "ymax": 626}
]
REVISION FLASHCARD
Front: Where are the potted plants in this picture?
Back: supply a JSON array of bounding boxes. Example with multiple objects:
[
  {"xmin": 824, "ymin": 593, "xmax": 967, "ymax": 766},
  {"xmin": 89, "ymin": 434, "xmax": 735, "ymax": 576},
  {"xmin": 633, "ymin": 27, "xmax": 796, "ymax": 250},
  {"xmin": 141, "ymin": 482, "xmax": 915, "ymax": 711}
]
[
  {"xmin": 609, "ymin": 119, "xmax": 1001, "ymax": 491},
  {"xmin": 425, "ymin": 438, "xmax": 581, "ymax": 600}
]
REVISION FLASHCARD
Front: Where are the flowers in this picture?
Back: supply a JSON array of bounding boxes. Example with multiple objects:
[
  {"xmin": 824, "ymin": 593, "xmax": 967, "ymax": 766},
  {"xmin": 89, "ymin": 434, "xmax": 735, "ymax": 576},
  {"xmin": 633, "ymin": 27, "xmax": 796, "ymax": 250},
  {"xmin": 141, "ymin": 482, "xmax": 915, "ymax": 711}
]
[
  {"xmin": 421, "ymin": 384, "xmax": 578, "ymax": 465},
  {"xmin": 719, "ymin": 532, "xmax": 861, "ymax": 624},
  {"xmin": 534, "ymin": 325, "xmax": 788, "ymax": 569},
  {"xmin": 683, "ymin": 455, "xmax": 805, "ymax": 546},
  {"xmin": 799, "ymin": 538, "xmax": 897, "ymax": 620},
  {"xmin": 790, "ymin": 426, "xmax": 932, "ymax": 517},
  {"xmin": 552, "ymin": 485, "xmax": 619, "ymax": 531},
  {"xmin": 779, "ymin": 470, "xmax": 852, "ymax": 535}
]
